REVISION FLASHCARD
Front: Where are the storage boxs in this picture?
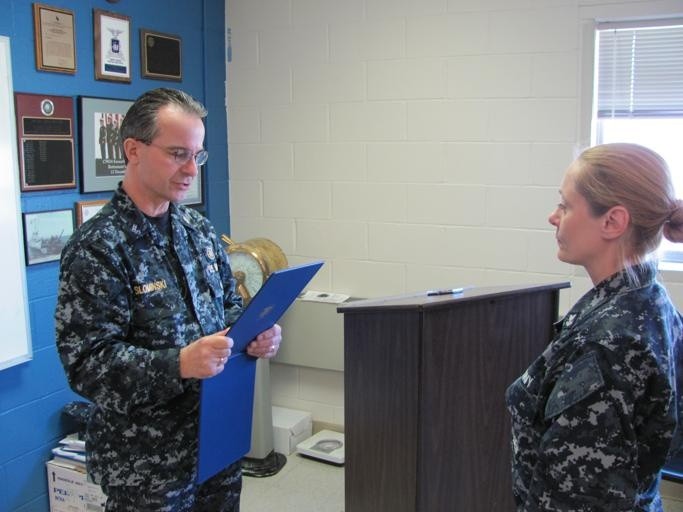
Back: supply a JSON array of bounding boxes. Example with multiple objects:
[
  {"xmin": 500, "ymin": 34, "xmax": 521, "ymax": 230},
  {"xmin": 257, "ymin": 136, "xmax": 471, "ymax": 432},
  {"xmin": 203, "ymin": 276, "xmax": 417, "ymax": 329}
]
[{"xmin": 45, "ymin": 459, "xmax": 109, "ymax": 512}]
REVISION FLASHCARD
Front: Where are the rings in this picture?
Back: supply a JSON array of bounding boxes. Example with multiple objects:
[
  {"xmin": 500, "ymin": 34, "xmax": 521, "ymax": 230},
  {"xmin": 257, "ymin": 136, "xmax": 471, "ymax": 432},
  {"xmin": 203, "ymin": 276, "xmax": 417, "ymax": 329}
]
[
  {"xmin": 268, "ymin": 344, "xmax": 277, "ymax": 355},
  {"xmin": 215, "ymin": 354, "xmax": 224, "ymax": 365}
]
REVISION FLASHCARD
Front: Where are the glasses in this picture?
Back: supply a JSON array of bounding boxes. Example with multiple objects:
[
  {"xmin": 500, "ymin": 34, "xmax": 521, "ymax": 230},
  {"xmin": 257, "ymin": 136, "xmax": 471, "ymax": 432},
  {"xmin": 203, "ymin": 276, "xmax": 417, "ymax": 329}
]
[{"xmin": 127, "ymin": 137, "xmax": 209, "ymax": 167}]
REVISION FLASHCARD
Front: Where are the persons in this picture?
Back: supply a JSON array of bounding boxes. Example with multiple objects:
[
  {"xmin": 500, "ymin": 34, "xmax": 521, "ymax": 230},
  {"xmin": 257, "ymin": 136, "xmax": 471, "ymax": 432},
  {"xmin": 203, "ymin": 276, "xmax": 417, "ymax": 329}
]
[
  {"xmin": 98, "ymin": 113, "xmax": 126, "ymax": 160},
  {"xmin": 54, "ymin": 87, "xmax": 283, "ymax": 510},
  {"xmin": 502, "ymin": 140, "xmax": 682, "ymax": 510}
]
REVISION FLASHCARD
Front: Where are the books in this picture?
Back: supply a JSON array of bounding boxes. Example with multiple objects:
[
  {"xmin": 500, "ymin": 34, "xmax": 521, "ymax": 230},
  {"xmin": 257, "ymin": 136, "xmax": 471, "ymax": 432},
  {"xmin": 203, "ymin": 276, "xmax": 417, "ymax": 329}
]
[{"xmin": 51, "ymin": 429, "xmax": 87, "ymax": 476}]
